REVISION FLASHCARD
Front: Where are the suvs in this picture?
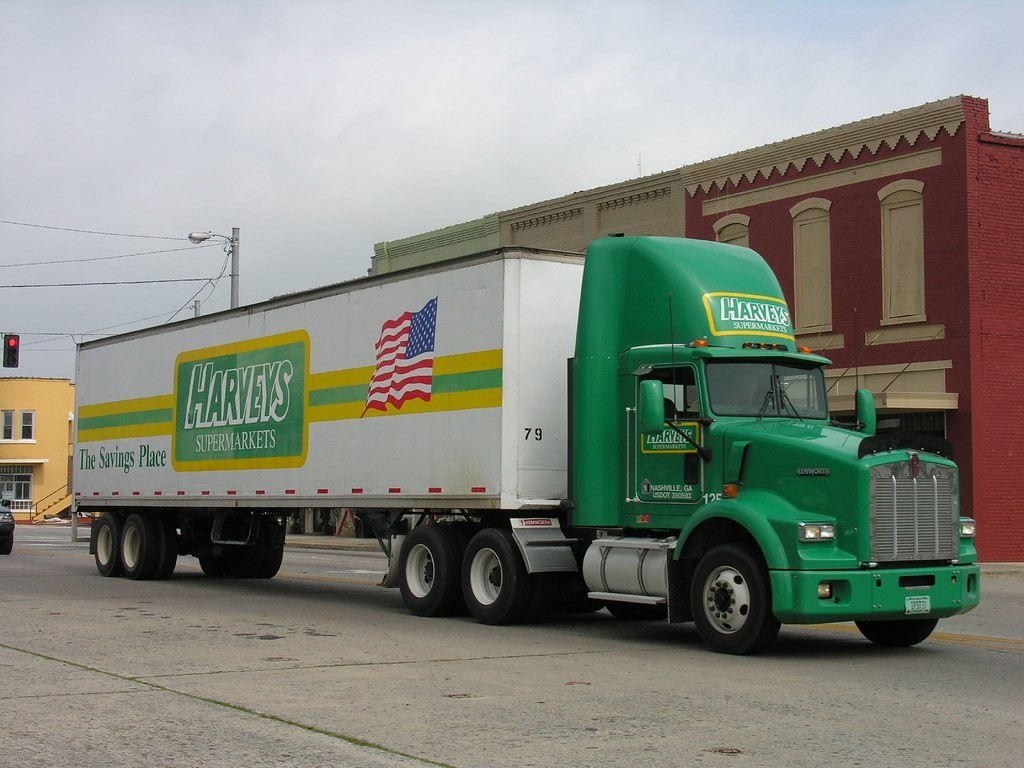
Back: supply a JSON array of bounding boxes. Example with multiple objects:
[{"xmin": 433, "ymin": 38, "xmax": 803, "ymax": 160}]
[{"xmin": 0, "ymin": 500, "xmax": 15, "ymax": 556}]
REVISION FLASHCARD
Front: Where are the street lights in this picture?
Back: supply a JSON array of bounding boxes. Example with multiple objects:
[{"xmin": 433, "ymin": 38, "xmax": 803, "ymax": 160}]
[{"xmin": 190, "ymin": 232, "xmax": 239, "ymax": 311}]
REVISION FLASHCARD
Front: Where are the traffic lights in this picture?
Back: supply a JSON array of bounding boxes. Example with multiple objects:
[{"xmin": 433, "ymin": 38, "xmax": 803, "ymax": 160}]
[{"xmin": 3, "ymin": 334, "xmax": 20, "ymax": 367}]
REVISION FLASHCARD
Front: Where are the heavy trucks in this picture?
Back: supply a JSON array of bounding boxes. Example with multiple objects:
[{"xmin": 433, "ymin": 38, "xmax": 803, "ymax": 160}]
[{"xmin": 72, "ymin": 234, "xmax": 984, "ymax": 655}]
